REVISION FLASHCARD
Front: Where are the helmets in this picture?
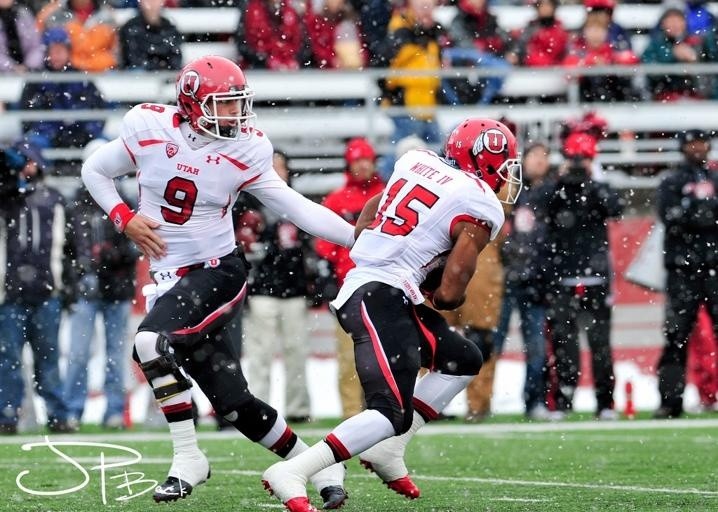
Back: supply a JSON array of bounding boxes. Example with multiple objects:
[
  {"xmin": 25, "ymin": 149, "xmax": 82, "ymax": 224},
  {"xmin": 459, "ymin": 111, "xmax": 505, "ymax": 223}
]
[
  {"xmin": 562, "ymin": 130, "xmax": 598, "ymax": 158},
  {"xmin": 345, "ymin": 140, "xmax": 376, "ymax": 162},
  {"xmin": 444, "ymin": 118, "xmax": 523, "ymax": 207},
  {"xmin": 177, "ymin": 59, "xmax": 256, "ymax": 142}
]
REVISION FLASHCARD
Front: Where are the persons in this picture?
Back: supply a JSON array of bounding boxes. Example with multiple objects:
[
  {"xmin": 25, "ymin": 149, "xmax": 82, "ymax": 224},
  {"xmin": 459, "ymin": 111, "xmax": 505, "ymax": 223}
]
[
  {"xmin": 238, "ymin": 0, "xmax": 405, "ymax": 68},
  {"xmin": 498, "ymin": 141, "xmax": 560, "ymax": 424},
  {"xmin": 445, "ymin": 1, "xmax": 564, "ymax": 67},
  {"xmin": 314, "ymin": 134, "xmax": 389, "ymax": 418},
  {"xmin": 559, "ymin": 0, "xmax": 718, "ymax": 104},
  {"xmin": 116, "ymin": 1, "xmax": 183, "ymax": 73},
  {"xmin": 261, "ymin": 116, "xmax": 521, "ymax": 512},
  {"xmin": 376, "ymin": 1, "xmax": 447, "ymax": 183},
  {"xmin": 234, "ymin": 148, "xmax": 310, "ymax": 424},
  {"xmin": 20, "ymin": 29, "xmax": 106, "ymax": 177},
  {"xmin": 62, "ymin": 138, "xmax": 140, "ymax": 435},
  {"xmin": 80, "ymin": 54, "xmax": 386, "ymax": 507},
  {"xmin": 651, "ymin": 128, "xmax": 717, "ymax": 421},
  {"xmin": 2, "ymin": 1, "xmax": 116, "ymax": 72},
  {"xmin": 0, "ymin": 131, "xmax": 78, "ymax": 437},
  {"xmin": 532, "ymin": 120, "xmax": 626, "ymax": 423}
]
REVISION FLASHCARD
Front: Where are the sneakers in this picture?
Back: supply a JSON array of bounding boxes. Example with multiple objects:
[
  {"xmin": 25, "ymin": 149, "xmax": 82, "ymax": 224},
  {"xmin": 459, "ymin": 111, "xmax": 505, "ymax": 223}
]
[
  {"xmin": 548, "ymin": 411, "xmax": 563, "ymax": 420},
  {"xmin": 599, "ymin": 408, "xmax": 616, "ymax": 418},
  {"xmin": 260, "ymin": 464, "xmax": 318, "ymax": 510},
  {"xmin": 313, "ymin": 463, "xmax": 349, "ymax": 512},
  {"xmin": 153, "ymin": 451, "xmax": 212, "ymax": 503},
  {"xmin": 358, "ymin": 448, "xmax": 419, "ymax": 499},
  {"xmin": 531, "ymin": 404, "xmax": 548, "ymax": 417}
]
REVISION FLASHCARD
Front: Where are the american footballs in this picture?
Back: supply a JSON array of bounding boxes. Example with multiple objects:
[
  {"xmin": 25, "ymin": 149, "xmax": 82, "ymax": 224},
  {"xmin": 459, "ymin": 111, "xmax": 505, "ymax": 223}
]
[{"xmin": 419, "ymin": 266, "xmax": 445, "ymax": 296}]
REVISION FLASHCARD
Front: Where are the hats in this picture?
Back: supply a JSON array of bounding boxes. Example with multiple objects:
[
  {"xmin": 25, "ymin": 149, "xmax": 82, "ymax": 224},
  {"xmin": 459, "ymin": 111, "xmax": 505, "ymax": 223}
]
[
  {"xmin": 680, "ymin": 130, "xmax": 714, "ymax": 146},
  {"xmin": 42, "ymin": 26, "xmax": 70, "ymax": 43}
]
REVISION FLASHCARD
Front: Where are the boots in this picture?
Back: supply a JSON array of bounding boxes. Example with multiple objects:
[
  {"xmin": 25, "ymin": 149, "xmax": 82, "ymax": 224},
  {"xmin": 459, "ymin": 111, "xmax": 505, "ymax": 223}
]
[{"xmin": 654, "ymin": 397, "xmax": 684, "ymax": 420}]
